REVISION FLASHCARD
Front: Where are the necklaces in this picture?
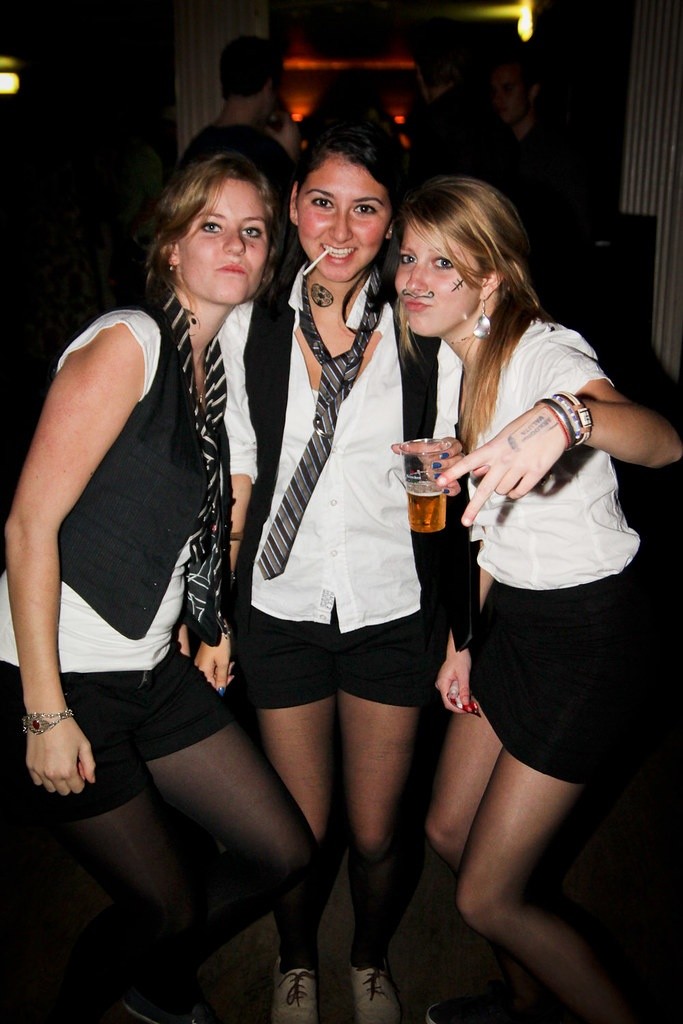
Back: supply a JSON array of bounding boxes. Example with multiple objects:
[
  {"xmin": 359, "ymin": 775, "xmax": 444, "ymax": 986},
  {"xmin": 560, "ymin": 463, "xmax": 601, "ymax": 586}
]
[{"xmin": 199, "ymin": 394, "xmax": 203, "ymax": 403}]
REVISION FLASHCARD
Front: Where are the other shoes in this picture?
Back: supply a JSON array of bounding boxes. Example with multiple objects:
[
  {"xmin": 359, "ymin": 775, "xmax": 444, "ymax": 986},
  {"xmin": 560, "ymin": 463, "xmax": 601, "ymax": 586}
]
[{"xmin": 121, "ymin": 987, "xmax": 225, "ymax": 1024}]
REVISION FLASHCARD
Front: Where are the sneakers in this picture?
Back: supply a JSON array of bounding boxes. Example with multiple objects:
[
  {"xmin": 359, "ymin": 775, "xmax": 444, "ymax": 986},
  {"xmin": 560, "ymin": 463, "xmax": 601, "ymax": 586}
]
[
  {"xmin": 348, "ymin": 952, "xmax": 400, "ymax": 1024},
  {"xmin": 270, "ymin": 955, "xmax": 317, "ymax": 1024},
  {"xmin": 425, "ymin": 982, "xmax": 565, "ymax": 1024}
]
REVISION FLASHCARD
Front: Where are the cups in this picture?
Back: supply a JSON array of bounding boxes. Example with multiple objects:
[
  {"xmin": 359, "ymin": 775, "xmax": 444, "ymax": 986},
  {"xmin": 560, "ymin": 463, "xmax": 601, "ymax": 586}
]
[{"xmin": 398, "ymin": 437, "xmax": 452, "ymax": 533}]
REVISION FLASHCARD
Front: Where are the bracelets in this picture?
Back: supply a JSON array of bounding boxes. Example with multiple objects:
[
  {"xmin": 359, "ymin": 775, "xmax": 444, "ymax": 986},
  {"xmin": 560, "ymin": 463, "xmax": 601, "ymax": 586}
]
[
  {"xmin": 22, "ymin": 710, "xmax": 72, "ymax": 734},
  {"xmin": 534, "ymin": 391, "xmax": 592, "ymax": 452}
]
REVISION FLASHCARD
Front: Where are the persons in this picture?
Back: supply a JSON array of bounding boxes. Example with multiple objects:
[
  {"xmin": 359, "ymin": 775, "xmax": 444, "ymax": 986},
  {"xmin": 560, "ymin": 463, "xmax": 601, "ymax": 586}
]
[
  {"xmin": 194, "ymin": 110, "xmax": 466, "ymax": 1024},
  {"xmin": 0, "ymin": 151, "xmax": 285, "ymax": 1024},
  {"xmin": 122, "ymin": 16, "xmax": 587, "ymax": 220},
  {"xmin": 394, "ymin": 177, "xmax": 682, "ymax": 1024}
]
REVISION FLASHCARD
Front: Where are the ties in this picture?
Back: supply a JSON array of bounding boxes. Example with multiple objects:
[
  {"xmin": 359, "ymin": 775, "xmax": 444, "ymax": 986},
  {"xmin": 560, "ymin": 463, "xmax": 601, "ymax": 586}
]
[
  {"xmin": 257, "ymin": 263, "xmax": 380, "ymax": 580},
  {"xmin": 155, "ymin": 282, "xmax": 229, "ymax": 644}
]
